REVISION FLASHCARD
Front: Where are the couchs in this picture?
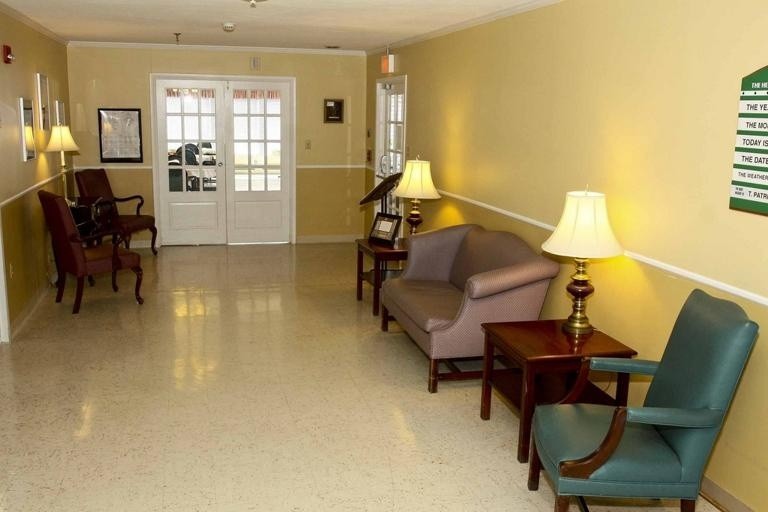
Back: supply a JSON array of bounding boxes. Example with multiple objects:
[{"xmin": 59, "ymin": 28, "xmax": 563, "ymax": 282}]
[{"xmin": 381, "ymin": 223, "xmax": 560, "ymax": 393}]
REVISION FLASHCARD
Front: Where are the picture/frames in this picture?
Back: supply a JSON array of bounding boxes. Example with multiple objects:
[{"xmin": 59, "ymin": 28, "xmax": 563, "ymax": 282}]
[
  {"xmin": 19, "ymin": 96, "xmax": 37, "ymax": 162},
  {"xmin": 368, "ymin": 212, "xmax": 402, "ymax": 245},
  {"xmin": 97, "ymin": 107, "xmax": 143, "ymax": 163},
  {"xmin": 36, "ymin": 72, "xmax": 51, "ymax": 131},
  {"xmin": 324, "ymin": 98, "xmax": 344, "ymax": 123},
  {"xmin": 56, "ymin": 100, "xmax": 65, "ymax": 125}
]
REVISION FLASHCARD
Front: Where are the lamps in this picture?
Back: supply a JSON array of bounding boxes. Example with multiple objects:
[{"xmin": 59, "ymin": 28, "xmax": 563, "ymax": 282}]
[
  {"xmin": 390, "ymin": 152, "xmax": 442, "ymax": 235},
  {"xmin": 45, "ymin": 122, "xmax": 81, "ymax": 208},
  {"xmin": 541, "ymin": 183, "xmax": 623, "ymax": 335}
]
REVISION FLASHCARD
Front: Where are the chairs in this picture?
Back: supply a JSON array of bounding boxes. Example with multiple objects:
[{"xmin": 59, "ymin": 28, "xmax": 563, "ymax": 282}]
[
  {"xmin": 75, "ymin": 168, "xmax": 158, "ymax": 255},
  {"xmin": 527, "ymin": 288, "xmax": 759, "ymax": 512},
  {"xmin": 37, "ymin": 190, "xmax": 144, "ymax": 314}
]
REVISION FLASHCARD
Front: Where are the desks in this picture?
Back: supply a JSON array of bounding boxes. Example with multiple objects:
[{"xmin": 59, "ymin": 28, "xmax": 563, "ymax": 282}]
[
  {"xmin": 480, "ymin": 319, "xmax": 638, "ymax": 463},
  {"xmin": 355, "ymin": 237, "xmax": 408, "ymax": 316},
  {"xmin": 66, "ymin": 196, "xmax": 103, "ymax": 286}
]
